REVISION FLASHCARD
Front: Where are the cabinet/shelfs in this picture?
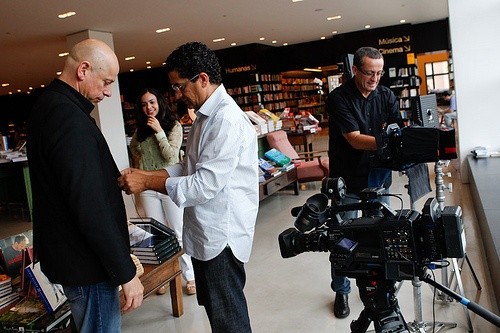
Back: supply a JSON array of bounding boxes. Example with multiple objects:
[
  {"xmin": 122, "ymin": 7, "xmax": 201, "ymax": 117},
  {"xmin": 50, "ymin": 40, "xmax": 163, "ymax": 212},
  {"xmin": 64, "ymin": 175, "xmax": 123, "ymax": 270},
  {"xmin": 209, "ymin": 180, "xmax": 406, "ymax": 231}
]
[{"xmin": 225, "ymin": 64, "xmax": 420, "ymax": 128}]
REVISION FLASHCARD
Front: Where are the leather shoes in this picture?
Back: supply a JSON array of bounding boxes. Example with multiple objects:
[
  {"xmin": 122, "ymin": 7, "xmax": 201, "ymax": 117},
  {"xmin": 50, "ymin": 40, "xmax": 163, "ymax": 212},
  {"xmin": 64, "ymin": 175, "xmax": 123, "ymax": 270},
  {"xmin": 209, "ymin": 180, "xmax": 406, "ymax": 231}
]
[{"xmin": 334, "ymin": 292, "xmax": 350, "ymax": 318}]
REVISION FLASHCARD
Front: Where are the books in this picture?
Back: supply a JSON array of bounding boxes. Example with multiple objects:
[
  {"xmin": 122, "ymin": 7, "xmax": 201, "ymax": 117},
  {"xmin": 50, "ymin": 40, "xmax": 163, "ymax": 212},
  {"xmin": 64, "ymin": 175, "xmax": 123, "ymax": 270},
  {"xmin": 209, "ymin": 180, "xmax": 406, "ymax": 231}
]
[{"xmin": 0, "ymin": 53, "xmax": 420, "ymax": 332}]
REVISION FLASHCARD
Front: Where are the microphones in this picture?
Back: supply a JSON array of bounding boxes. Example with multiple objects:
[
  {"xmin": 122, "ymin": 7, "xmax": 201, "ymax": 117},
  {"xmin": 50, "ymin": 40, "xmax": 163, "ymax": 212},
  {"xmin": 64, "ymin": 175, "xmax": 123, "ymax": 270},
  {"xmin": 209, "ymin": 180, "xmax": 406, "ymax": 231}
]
[{"xmin": 291, "ymin": 202, "xmax": 375, "ymax": 216}]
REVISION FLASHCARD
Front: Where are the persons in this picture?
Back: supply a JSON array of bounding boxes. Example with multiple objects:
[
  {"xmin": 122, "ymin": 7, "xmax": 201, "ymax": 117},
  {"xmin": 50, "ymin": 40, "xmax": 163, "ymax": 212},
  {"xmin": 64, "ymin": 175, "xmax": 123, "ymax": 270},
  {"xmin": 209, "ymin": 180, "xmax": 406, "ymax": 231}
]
[
  {"xmin": 326, "ymin": 47, "xmax": 405, "ymax": 318},
  {"xmin": 129, "ymin": 89, "xmax": 196, "ymax": 295},
  {"xmin": 26, "ymin": 38, "xmax": 144, "ymax": 333},
  {"xmin": 440, "ymin": 90, "xmax": 457, "ymax": 128},
  {"xmin": 5, "ymin": 234, "xmax": 28, "ymax": 278},
  {"xmin": 117, "ymin": 42, "xmax": 259, "ymax": 333}
]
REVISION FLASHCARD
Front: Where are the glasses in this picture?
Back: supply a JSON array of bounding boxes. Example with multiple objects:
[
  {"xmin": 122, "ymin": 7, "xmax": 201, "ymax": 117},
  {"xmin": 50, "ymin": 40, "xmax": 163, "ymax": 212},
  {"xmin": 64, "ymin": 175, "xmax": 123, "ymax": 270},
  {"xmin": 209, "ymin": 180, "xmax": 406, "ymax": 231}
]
[
  {"xmin": 171, "ymin": 72, "xmax": 202, "ymax": 93},
  {"xmin": 355, "ymin": 66, "xmax": 385, "ymax": 78}
]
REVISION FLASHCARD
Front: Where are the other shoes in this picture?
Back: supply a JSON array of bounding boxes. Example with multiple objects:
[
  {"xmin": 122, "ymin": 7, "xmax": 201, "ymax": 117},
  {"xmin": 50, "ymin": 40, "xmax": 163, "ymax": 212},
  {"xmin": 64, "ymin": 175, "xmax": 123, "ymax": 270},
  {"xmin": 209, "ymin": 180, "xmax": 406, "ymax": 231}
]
[
  {"xmin": 186, "ymin": 280, "xmax": 197, "ymax": 295},
  {"xmin": 157, "ymin": 282, "xmax": 169, "ymax": 295}
]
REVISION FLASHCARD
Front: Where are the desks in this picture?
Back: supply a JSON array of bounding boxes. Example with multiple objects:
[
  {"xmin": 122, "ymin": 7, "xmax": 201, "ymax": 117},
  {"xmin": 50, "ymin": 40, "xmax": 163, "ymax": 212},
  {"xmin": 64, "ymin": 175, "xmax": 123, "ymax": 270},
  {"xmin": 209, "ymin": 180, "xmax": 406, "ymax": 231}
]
[
  {"xmin": 259, "ymin": 163, "xmax": 301, "ymax": 195},
  {"xmin": 258, "ymin": 128, "xmax": 289, "ymax": 157},
  {"xmin": 0, "ymin": 160, "xmax": 33, "ymax": 222},
  {"xmin": 0, "ymin": 249, "xmax": 185, "ymax": 319},
  {"xmin": 286, "ymin": 130, "xmax": 315, "ymax": 162}
]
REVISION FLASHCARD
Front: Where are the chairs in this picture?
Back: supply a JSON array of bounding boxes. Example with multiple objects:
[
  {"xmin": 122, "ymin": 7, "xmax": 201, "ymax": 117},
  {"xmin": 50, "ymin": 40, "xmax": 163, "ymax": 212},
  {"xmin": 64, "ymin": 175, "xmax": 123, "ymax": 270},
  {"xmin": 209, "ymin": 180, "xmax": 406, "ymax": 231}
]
[{"xmin": 266, "ymin": 130, "xmax": 329, "ymax": 183}]
